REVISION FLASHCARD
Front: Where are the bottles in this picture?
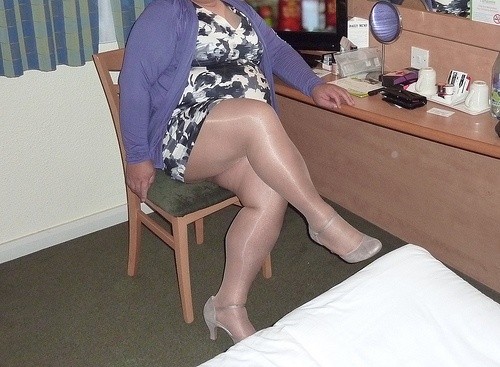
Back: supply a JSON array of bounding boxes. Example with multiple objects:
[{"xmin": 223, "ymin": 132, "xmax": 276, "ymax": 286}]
[
  {"xmin": 261, "ymin": 7, "xmax": 273, "ymax": 28},
  {"xmin": 491, "ymin": 51, "xmax": 500, "ymax": 118},
  {"xmin": 278, "ymin": 0, "xmax": 301, "ymax": 31}
]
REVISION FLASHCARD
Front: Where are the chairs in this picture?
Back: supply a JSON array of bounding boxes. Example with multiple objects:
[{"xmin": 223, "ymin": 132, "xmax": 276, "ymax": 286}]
[{"xmin": 92, "ymin": 47, "xmax": 272, "ymax": 324}]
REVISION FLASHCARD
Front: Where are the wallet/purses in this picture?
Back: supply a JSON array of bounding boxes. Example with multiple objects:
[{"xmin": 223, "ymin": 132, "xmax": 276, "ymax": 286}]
[{"xmin": 382, "ymin": 88, "xmax": 427, "ymax": 110}]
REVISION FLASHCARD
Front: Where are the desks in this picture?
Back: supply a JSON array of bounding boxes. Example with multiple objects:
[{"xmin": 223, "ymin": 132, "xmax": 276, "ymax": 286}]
[{"xmin": 268, "ymin": 66, "xmax": 500, "ymax": 295}]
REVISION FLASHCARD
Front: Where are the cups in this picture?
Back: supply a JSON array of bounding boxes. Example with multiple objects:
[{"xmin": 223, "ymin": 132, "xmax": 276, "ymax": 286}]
[
  {"xmin": 465, "ymin": 80, "xmax": 489, "ymax": 112},
  {"xmin": 415, "ymin": 67, "xmax": 436, "ymax": 93},
  {"xmin": 301, "ymin": 0, "xmax": 320, "ymax": 32}
]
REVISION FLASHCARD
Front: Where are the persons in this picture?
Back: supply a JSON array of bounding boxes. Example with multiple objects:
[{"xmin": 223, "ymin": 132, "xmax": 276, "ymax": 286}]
[{"xmin": 119, "ymin": 0, "xmax": 383, "ymax": 344}]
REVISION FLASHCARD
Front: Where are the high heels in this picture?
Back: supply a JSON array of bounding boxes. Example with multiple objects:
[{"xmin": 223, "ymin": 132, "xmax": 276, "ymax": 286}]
[
  {"xmin": 202, "ymin": 296, "xmax": 256, "ymax": 345},
  {"xmin": 307, "ymin": 206, "xmax": 383, "ymax": 264}
]
